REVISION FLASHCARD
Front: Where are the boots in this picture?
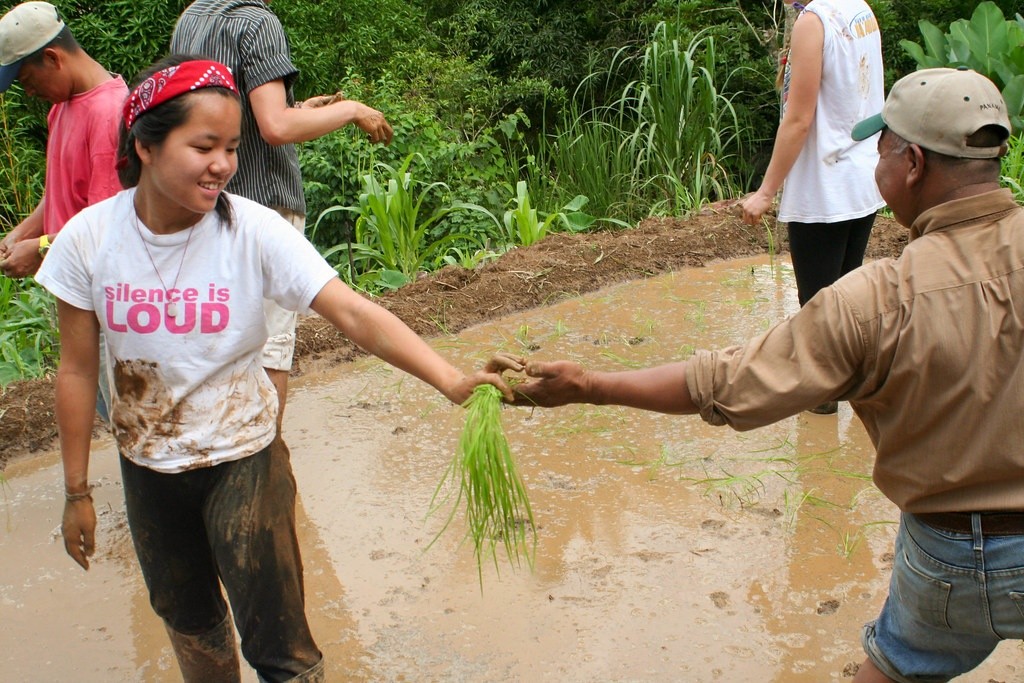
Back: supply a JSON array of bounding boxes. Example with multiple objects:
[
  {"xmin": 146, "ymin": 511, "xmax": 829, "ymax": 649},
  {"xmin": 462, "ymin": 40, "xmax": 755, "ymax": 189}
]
[
  {"xmin": 164, "ymin": 601, "xmax": 241, "ymax": 683},
  {"xmin": 257, "ymin": 651, "xmax": 326, "ymax": 683}
]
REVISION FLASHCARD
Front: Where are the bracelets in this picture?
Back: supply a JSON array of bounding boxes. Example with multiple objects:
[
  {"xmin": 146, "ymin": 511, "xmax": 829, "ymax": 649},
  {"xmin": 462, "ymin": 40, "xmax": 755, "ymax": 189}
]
[{"xmin": 66, "ymin": 489, "xmax": 94, "ymax": 501}]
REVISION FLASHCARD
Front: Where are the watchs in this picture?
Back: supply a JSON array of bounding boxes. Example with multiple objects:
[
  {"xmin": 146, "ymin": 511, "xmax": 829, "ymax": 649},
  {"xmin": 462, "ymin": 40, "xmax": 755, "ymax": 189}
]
[{"xmin": 38, "ymin": 234, "xmax": 51, "ymax": 258}]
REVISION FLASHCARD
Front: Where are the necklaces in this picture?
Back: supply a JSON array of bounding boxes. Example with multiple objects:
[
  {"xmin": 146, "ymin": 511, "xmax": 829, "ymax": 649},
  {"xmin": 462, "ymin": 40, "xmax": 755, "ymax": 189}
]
[{"xmin": 137, "ymin": 212, "xmax": 194, "ymax": 316}]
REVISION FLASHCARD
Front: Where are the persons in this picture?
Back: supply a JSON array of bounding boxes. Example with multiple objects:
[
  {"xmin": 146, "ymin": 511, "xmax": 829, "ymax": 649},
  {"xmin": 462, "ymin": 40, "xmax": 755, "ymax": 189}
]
[
  {"xmin": 736, "ymin": 0, "xmax": 887, "ymax": 414},
  {"xmin": 0, "ymin": 1, "xmax": 131, "ymax": 426},
  {"xmin": 501, "ymin": 65, "xmax": 1024, "ymax": 683},
  {"xmin": 168, "ymin": 1, "xmax": 384, "ymax": 438},
  {"xmin": 34, "ymin": 53, "xmax": 529, "ymax": 682}
]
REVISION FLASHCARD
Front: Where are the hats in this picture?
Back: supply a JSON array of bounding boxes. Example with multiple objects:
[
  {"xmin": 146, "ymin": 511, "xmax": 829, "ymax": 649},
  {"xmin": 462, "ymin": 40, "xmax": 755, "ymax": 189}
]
[
  {"xmin": 0, "ymin": 1, "xmax": 65, "ymax": 93},
  {"xmin": 851, "ymin": 66, "xmax": 1012, "ymax": 158}
]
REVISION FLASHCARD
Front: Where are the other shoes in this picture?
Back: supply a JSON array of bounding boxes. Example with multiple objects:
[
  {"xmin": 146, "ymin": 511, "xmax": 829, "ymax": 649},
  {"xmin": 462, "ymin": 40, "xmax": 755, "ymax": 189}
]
[{"xmin": 809, "ymin": 400, "xmax": 837, "ymax": 415}]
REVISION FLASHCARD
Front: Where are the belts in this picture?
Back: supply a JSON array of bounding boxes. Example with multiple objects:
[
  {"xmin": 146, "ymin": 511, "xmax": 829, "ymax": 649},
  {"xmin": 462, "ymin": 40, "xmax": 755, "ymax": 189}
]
[{"xmin": 915, "ymin": 511, "xmax": 1024, "ymax": 535}]
